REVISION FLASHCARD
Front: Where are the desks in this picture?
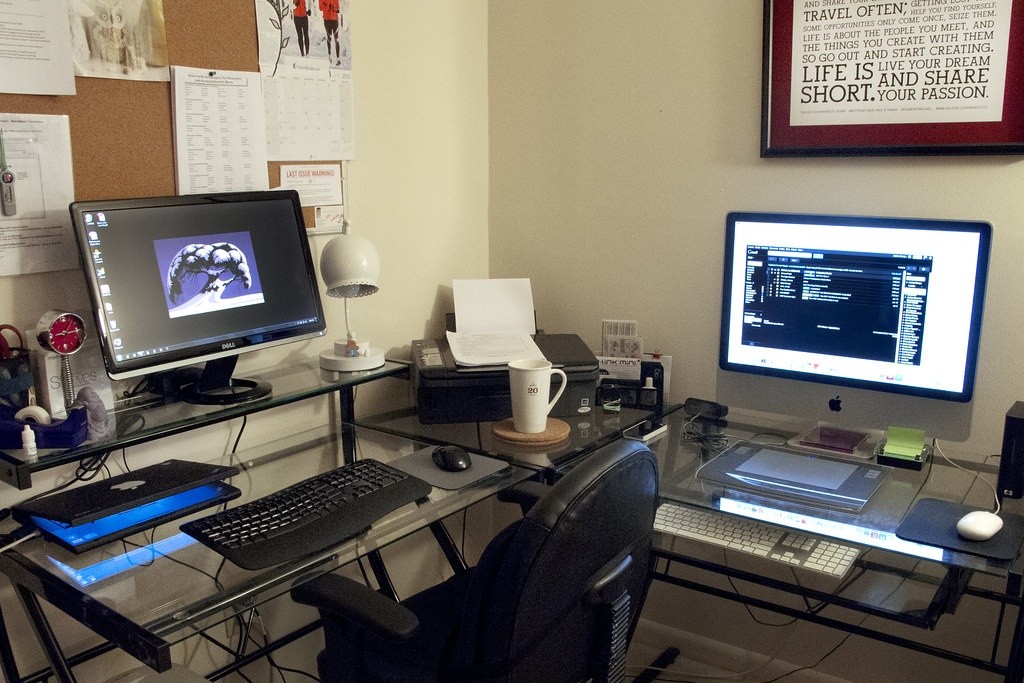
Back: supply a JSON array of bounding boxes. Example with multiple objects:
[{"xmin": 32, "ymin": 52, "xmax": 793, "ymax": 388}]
[
  {"xmin": 546, "ymin": 403, "xmax": 1024, "ymax": 683},
  {"xmin": 350, "ymin": 394, "xmax": 676, "ymax": 573},
  {"xmin": 0, "ymin": 420, "xmax": 538, "ymax": 683}
]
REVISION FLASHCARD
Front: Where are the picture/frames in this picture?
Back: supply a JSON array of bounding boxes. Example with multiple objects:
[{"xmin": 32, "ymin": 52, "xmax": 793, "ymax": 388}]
[{"xmin": 758, "ymin": 0, "xmax": 1024, "ymax": 160}]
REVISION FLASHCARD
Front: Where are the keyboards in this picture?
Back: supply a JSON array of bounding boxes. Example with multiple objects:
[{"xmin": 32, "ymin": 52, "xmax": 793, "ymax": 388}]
[
  {"xmin": 177, "ymin": 458, "xmax": 431, "ymax": 569},
  {"xmin": 653, "ymin": 503, "xmax": 861, "ymax": 579}
]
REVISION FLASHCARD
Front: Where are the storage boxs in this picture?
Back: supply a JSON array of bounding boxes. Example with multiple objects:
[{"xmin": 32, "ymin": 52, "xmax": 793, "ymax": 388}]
[{"xmin": 23, "ymin": 329, "xmax": 115, "ymax": 419}]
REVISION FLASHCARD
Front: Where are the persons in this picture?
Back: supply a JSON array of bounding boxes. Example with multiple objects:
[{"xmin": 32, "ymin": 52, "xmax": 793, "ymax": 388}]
[
  {"xmin": 72, "ymin": 387, "xmax": 110, "ymax": 442},
  {"xmin": 319, "ymin": 0, "xmax": 341, "ymax": 66},
  {"xmin": 289, "ymin": 0, "xmax": 311, "ymax": 56}
]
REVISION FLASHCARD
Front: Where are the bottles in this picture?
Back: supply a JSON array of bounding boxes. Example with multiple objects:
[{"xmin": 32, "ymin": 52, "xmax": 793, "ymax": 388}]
[{"xmin": 21, "ymin": 424, "xmax": 38, "ymax": 457}]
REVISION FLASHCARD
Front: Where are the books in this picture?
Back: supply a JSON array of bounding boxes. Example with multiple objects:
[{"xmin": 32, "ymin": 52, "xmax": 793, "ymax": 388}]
[{"xmin": 799, "ymin": 426, "xmax": 872, "ymax": 454}]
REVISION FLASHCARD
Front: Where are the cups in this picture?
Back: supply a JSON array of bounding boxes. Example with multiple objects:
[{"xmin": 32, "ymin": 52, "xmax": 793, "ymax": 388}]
[{"xmin": 507, "ymin": 357, "xmax": 568, "ymax": 434}]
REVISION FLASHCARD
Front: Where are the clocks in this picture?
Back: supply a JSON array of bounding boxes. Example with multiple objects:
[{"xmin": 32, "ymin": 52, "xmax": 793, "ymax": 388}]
[{"xmin": 36, "ymin": 308, "xmax": 88, "ymax": 409}]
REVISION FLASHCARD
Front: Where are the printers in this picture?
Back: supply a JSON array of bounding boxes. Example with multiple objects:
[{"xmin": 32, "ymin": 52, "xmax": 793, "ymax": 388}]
[{"xmin": 409, "ymin": 307, "xmax": 600, "ymax": 424}]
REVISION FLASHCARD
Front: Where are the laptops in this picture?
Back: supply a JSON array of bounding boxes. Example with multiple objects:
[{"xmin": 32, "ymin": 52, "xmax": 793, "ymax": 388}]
[{"xmin": 11, "ymin": 460, "xmax": 243, "ymax": 554}]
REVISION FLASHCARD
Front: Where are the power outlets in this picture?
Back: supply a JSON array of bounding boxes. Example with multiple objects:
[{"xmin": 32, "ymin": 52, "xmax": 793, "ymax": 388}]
[{"xmin": 224, "ymin": 577, "xmax": 254, "ymax": 637}]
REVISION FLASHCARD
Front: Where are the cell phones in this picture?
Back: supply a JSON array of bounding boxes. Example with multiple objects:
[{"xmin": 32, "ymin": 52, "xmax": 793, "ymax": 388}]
[{"xmin": 623, "ymin": 420, "xmax": 667, "ymax": 442}]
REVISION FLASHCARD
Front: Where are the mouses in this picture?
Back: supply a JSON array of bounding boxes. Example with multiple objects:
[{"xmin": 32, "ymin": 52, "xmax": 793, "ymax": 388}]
[
  {"xmin": 432, "ymin": 445, "xmax": 472, "ymax": 473},
  {"xmin": 955, "ymin": 511, "xmax": 1004, "ymax": 540}
]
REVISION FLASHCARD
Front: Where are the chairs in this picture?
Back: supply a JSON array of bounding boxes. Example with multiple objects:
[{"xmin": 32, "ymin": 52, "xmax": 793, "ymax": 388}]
[{"xmin": 290, "ymin": 438, "xmax": 662, "ymax": 683}]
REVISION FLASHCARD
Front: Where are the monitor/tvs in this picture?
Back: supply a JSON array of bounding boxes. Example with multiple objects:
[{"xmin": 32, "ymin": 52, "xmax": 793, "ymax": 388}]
[
  {"xmin": 69, "ymin": 190, "xmax": 329, "ymax": 405},
  {"xmin": 712, "ymin": 211, "xmax": 993, "ymax": 457}
]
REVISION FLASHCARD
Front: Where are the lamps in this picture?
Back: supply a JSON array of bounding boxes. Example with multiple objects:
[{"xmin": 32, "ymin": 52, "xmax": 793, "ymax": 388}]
[{"xmin": 318, "ymin": 218, "xmax": 385, "ymax": 373}]
